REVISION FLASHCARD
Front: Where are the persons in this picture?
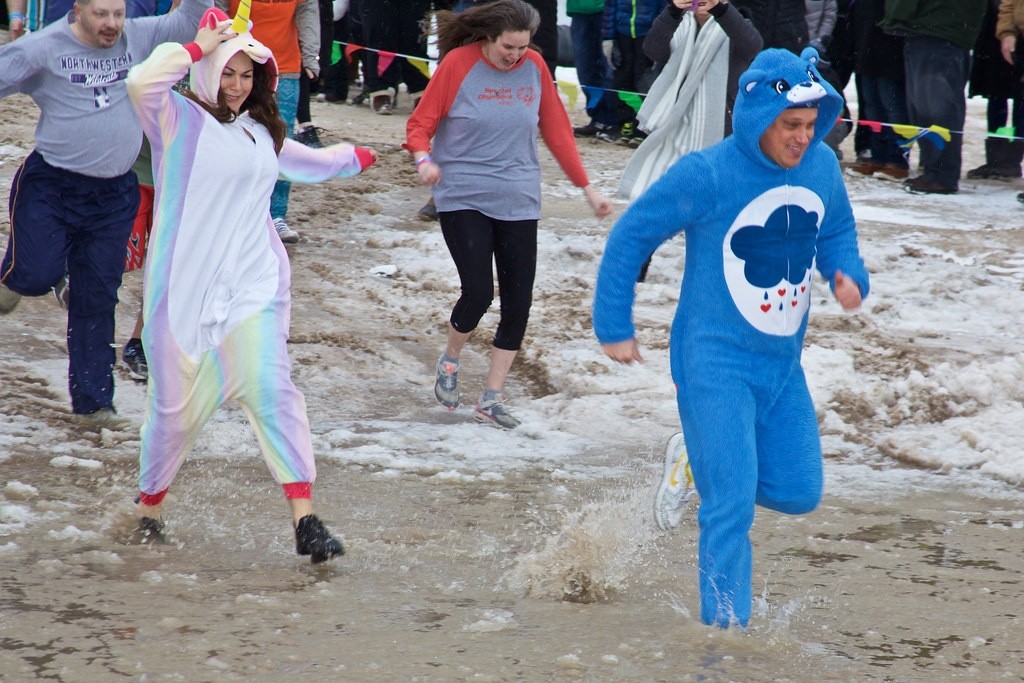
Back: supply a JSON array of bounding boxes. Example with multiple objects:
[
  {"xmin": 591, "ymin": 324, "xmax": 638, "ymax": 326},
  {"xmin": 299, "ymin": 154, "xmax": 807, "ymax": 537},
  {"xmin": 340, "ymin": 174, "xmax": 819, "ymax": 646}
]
[
  {"xmin": 593, "ymin": 46, "xmax": 870, "ymax": 630},
  {"xmin": 125, "ymin": 0, "xmax": 321, "ymax": 243},
  {"xmin": 838, "ymin": 0, "xmax": 1024, "ymax": 205},
  {"xmin": 402, "ymin": 0, "xmax": 613, "ymax": 431},
  {"xmin": 128, "ymin": 0, "xmax": 380, "ymax": 564},
  {"xmin": 7, "ymin": 0, "xmax": 72, "ymax": 41},
  {"xmin": 0, "ymin": 0, "xmax": 213, "ymax": 429},
  {"xmin": 614, "ymin": 0, "xmax": 763, "ymax": 282},
  {"xmin": 565, "ymin": 0, "xmax": 665, "ymax": 148},
  {"xmin": 435, "ymin": 0, "xmax": 559, "ymax": 93},
  {"xmin": 314, "ymin": 0, "xmax": 432, "ymax": 113},
  {"xmin": 733, "ymin": 0, "xmax": 853, "ymax": 161}
]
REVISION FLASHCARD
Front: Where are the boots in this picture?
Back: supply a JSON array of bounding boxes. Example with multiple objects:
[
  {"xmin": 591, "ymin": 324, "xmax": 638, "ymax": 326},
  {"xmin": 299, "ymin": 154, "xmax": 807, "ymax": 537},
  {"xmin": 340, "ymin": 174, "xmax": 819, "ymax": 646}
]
[{"xmin": 966, "ymin": 138, "xmax": 1023, "ymax": 180}]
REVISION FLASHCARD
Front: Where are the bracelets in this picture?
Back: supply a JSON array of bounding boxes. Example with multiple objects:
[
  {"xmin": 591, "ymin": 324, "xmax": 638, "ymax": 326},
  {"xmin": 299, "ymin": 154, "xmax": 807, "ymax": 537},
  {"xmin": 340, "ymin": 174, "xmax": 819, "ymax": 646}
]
[
  {"xmin": 416, "ymin": 156, "xmax": 432, "ymax": 167},
  {"xmin": 10, "ymin": 13, "xmax": 25, "ymax": 20}
]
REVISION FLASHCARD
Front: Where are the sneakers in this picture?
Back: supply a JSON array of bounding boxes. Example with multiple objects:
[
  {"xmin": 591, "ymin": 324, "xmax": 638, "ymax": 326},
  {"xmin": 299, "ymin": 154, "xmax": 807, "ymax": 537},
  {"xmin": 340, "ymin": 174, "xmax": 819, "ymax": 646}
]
[
  {"xmin": 293, "ymin": 513, "xmax": 344, "ymax": 562},
  {"xmin": 79, "ymin": 405, "xmax": 133, "ymax": 432},
  {"xmin": 654, "ymin": 433, "xmax": 696, "ymax": 531},
  {"xmin": 473, "ymin": 395, "xmax": 521, "ymax": 430},
  {"xmin": 846, "ymin": 161, "xmax": 876, "ymax": 177},
  {"xmin": 0, "ymin": 282, "xmax": 22, "ymax": 314},
  {"xmin": 118, "ymin": 339, "xmax": 148, "ymax": 380},
  {"xmin": 50, "ymin": 275, "xmax": 70, "ymax": 310},
  {"xmin": 905, "ymin": 174, "xmax": 957, "ymax": 195},
  {"xmin": 572, "ymin": 120, "xmax": 646, "ymax": 147},
  {"xmin": 273, "ymin": 216, "xmax": 300, "ymax": 244},
  {"xmin": 296, "ymin": 125, "xmax": 329, "ymax": 149},
  {"xmin": 873, "ymin": 165, "xmax": 909, "ymax": 181},
  {"xmin": 434, "ymin": 352, "xmax": 461, "ymax": 411},
  {"xmin": 141, "ymin": 516, "xmax": 164, "ymax": 544}
]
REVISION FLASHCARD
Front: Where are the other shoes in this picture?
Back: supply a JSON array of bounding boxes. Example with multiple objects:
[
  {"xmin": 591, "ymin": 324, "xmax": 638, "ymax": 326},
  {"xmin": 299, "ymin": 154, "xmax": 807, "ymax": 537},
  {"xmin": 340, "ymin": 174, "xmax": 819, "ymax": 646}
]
[{"xmin": 418, "ymin": 203, "xmax": 437, "ymax": 220}]
